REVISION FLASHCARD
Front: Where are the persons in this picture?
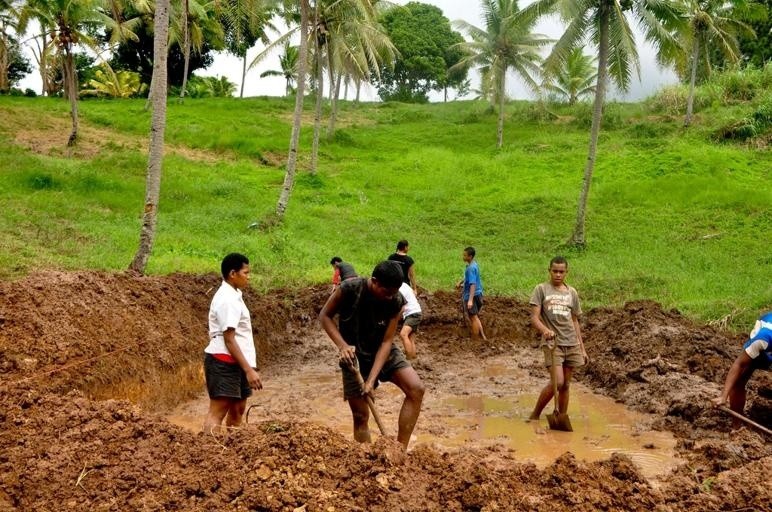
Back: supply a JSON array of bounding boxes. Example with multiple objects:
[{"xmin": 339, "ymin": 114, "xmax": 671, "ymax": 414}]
[
  {"xmin": 387, "ymin": 239, "xmax": 419, "ymax": 296},
  {"xmin": 454, "ymin": 245, "xmax": 483, "ymax": 338},
  {"xmin": 397, "ymin": 279, "xmax": 423, "ymax": 359},
  {"xmin": 317, "ymin": 258, "xmax": 427, "ymax": 453},
  {"xmin": 526, "ymin": 254, "xmax": 588, "ymax": 421},
  {"xmin": 709, "ymin": 309, "xmax": 771, "ymax": 439},
  {"xmin": 203, "ymin": 251, "xmax": 265, "ymax": 432},
  {"xmin": 329, "ymin": 256, "xmax": 358, "ymax": 292}
]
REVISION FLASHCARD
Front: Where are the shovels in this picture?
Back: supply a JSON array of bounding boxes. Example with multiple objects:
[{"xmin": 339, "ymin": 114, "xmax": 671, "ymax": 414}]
[
  {"xmin": 347, "ymin": 352, "xmax": 407, "ymax": 467},
  {"xmin": 545, "ymin": 333, "xmax": 573, "ymax": 431},
  {"xmin": 458, "ymin": 285, "xmax": 470, "ymax": 338}
]
